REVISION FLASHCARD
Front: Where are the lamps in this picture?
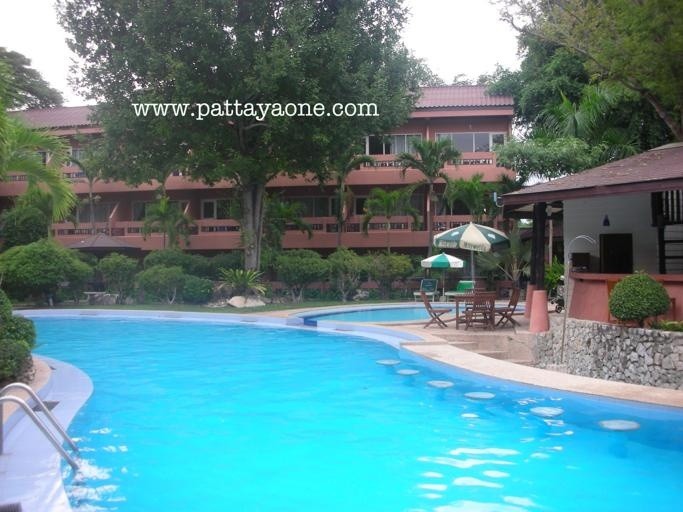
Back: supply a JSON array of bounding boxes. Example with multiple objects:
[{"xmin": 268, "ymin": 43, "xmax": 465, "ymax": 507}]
[
  {"xmin": 602, "ymin": 192, "xmax": 610, "ymax": 226},
  {"xmin": 494, "ymin": 192, "xmax": 504, "ymax": 208}
]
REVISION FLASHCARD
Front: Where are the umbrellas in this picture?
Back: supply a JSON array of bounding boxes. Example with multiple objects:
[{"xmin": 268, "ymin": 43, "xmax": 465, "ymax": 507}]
[
  {"xmin": 432, "ymin": 219, "xmax": 510, "ymax": 288},
  {"xmin": 420, "ymin": 251, "xmax": 469, "ymax": 295}
]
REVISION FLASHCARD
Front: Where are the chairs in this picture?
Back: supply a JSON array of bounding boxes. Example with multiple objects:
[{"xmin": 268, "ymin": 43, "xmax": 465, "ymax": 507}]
[{"xmin": 414, "ymin": 279, "xmax": 521, "ymax": 331}]
